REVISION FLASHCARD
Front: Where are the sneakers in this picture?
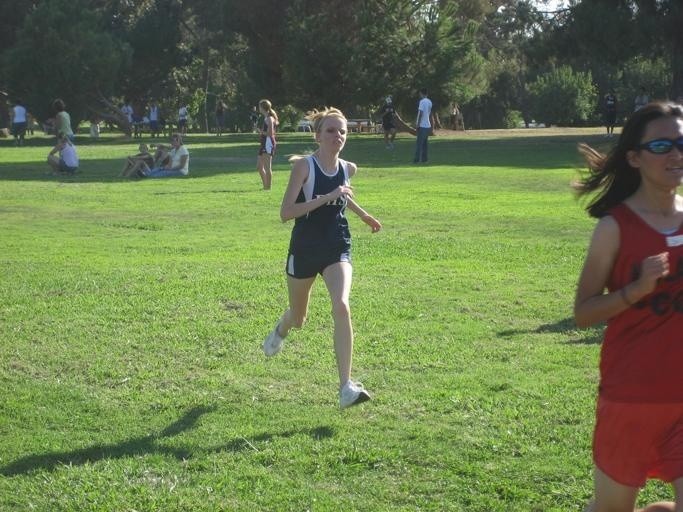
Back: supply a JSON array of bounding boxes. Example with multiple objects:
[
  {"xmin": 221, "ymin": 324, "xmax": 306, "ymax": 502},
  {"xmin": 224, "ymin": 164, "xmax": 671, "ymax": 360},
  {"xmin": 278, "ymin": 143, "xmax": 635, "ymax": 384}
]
[
  {"xmin": 339, "ymin": 380, "xmax": 371, "ymax": 409},
  {"xmin": 262, "ymin": 318, "xmax": 293, "ymax": 357}
]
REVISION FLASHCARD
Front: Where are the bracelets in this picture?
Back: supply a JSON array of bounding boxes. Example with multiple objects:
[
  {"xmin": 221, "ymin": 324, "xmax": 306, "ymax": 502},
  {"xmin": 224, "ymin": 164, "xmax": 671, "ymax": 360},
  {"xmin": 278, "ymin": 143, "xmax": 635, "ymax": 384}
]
[{"xmin": 620, "ymin": 286, "xmax": 634, "ymax": 308}]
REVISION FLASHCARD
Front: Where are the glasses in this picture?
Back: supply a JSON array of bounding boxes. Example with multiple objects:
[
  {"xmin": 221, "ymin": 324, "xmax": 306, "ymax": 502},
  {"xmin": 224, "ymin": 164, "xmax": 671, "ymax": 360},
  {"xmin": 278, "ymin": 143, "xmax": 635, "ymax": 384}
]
[{"xmin": 632, "ymin": 136, "xmax": 682, "ymax": 153}]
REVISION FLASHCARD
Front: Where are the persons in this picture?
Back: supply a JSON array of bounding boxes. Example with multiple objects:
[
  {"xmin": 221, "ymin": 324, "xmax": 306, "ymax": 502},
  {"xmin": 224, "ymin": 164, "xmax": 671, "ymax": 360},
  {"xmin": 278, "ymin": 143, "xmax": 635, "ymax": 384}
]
[
  {"xmin": 566, "ymin": 101, "xmax": 683, "ymax": 511},
  {"xmin": 248, "ymin": 105, "xmax": 261, "ymax": 134},
  {"xmin": 603, "ymin": 87, "xmax": 618, "ymax": 138},
  {"xmin": 256, "ymin": 99, "xmax": 280, "ymax": 191},
  {"xmin": 633, "ymin": 87, "xmax": 648, "ymax": 112},
  {"xmin": 260, "ymin": 106, "xmax": 383, "ymax": 409},
  {"xmin": 381, "ymin": 97, "xmax": 397, "ymax": 151},
  {"xmin": 411, "ymin": 88, "xmax": 433, "ymax": 163},
  {"xmin": 450, "ymin": 104, "xmax": 458, "ymax": 130},
  {"xmin": 0, "ymin": 90, "xmax": 230, "ymax": 180}
]
